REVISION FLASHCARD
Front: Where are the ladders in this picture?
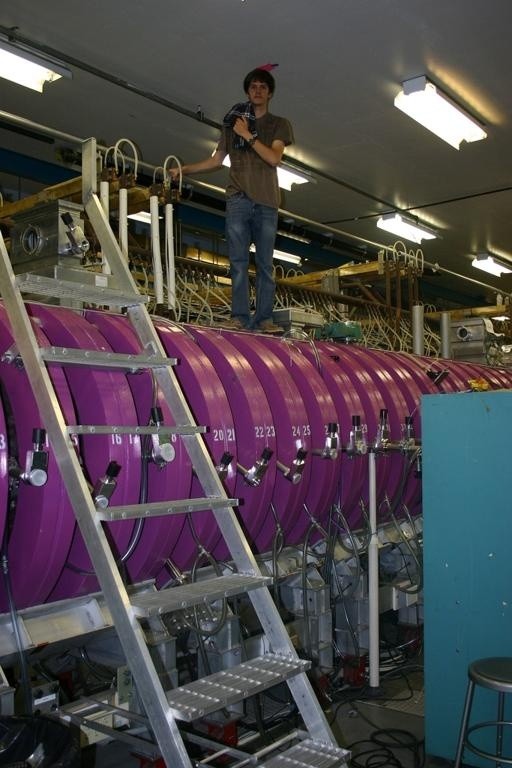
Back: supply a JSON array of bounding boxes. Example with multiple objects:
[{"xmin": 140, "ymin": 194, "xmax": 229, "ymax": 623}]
[{"xmin": 0, "ymin": 186, "xmax": 352, "ymax": 766}]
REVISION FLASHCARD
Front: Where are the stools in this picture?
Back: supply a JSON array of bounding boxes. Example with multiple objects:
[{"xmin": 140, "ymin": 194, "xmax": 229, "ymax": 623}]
[{"xmin": 455, "ymin": 656, "xmax": 512, "ymax": 768}]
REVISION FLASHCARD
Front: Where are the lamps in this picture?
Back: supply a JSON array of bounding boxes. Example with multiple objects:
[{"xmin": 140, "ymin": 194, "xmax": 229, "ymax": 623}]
[
  {"xmin": 250, "ymin": 243, "xmax": 308, "ymax": 265},
  {"xmin": 377, "ymin": 212, "xmax": 444, "ymax": 244},
  {"xmin": 394, "ymin": 76, "xmax": 487, "ymax": 150},
  {"xmin": 128, "ymin": 209, "xmax": 163, "ymax": 224},
  {"xmin": 210, "ymin": 142, "xmax": 318, "ymax": 191},
  {"xmin": 0, "ymin": 32, "xmax": 72, "ymax": 94},
  {"xmin": 472, "ymin": 253, "xmax": 512, "ymax": 277}
]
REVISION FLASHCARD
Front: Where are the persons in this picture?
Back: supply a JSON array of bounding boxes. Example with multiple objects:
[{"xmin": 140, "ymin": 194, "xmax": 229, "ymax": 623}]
[{"xmin": 167, "ymin": 66, "xmax": 296, "ymax": 337}]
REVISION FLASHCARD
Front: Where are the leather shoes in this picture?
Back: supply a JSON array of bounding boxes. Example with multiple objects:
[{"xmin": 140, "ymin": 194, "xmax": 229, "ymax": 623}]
[
  {"xmin": 257, "ymin": 320, "xmax": 285, "ymax": 333},
  {"xmin": 215, "ymin": 318, "xmax": 252, "ymax": 333}
]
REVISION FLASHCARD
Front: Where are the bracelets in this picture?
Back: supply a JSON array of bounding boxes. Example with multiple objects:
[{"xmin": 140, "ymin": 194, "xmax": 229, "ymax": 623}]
[{"xmin": 247, "ymin": 137, "xmax": 258, "ymax": 148}]
